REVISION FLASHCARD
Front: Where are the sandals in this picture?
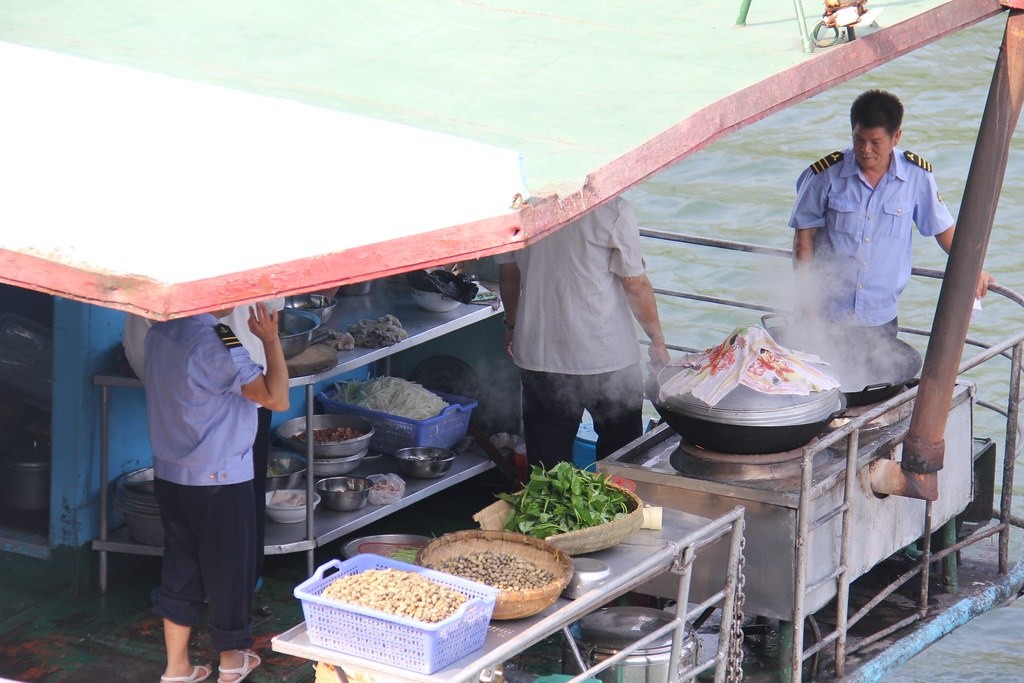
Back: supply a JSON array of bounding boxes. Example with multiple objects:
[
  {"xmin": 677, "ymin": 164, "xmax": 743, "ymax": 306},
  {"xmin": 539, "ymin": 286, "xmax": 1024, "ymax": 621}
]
[
  {"xmin": 216, "ymin": 646, "xmax": 262, "ymax": 683},
  {"xmin": 160, "ymin": 665, "xmax": 212, "ymax": 683}
]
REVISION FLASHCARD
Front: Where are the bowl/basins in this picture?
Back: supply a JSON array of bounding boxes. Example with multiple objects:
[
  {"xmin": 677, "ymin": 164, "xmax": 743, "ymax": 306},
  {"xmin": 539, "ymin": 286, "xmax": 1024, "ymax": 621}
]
[
  {"xmin": 336, "ymin": 280, "xmax": 375, "ymax": 296},
  {"xmin": 266, "ymin": 452, "xmax": 307, "ymax": 490},
  {"xmin": 304, "ymin": 448, "xmax": 369, "ymax": 476},
  {"xmin": 269, "ymin": 308, "xmax": 321, "ymax": 360},
  {"xmin": 284, "ymin": 293, "xmax": 339, "ymax": 325},
  {"xmin": 276, "ymin": 414, "xmax": 375, "ymax": 457},
  {"xmin": 265, "ymin": 489, "xmax": 321, "ymax": 523},
  {"xmin": 396, "ymin": 447, "xmax": 456, "ymax": 478},
  {"xmin": 112, "ymin": 466, "xmax": 164, "ymax": 546},
  {"xmin": 341, "ymin": 534, "xmax": 432, "ymax": 558},
  {"xmin": 410, "ymin": 288, "xmax": 461, "ymax": 312},
  {"xmin": 315, "ymin": 477, "xmax": 376, "ymax": 511},
  {"xmin": 560, "ymin": 557, "xmax": 611, "ymax": 599}
]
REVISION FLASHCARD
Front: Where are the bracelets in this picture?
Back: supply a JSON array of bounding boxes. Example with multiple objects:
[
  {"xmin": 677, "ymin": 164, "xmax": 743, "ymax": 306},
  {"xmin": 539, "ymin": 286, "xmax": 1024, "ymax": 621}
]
[{"xmin": 502, "ymin": 319, "xmax": 515, "ymax": 331}]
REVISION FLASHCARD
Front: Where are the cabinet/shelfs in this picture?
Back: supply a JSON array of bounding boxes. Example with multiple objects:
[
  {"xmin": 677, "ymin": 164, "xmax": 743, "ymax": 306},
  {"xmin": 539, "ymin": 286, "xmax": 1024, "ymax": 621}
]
[{"xmin": 91, "ymin": 277, "xmax": 517, "ymax": 597}]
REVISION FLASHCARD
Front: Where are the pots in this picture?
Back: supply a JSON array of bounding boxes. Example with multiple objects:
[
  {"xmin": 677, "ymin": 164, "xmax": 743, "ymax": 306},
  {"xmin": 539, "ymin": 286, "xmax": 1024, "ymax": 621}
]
[
  {"xmin": 0, "ymin": 440, "xmax": 51, "ymax": 511},
  {"xmin": 761, "ymin": 314, "xmax": 922, "ymax": 407},
  {"xmin": 645, "ymin": 366, "xmax": 849, "ymax": 455}
]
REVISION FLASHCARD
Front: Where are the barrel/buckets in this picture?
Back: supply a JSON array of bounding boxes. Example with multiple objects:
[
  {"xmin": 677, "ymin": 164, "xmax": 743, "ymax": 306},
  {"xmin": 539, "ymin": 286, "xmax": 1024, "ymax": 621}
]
[
  {"xmin": 514, "ymin": 444, "xmax": 530, "ymax": 492},
  {"xmin": 572, "ymin": 422, "xmax": 599, "ymax": 473},
  {"xmin": 561, "ymin": 607, "xmax": 704, "ymax": 683}
]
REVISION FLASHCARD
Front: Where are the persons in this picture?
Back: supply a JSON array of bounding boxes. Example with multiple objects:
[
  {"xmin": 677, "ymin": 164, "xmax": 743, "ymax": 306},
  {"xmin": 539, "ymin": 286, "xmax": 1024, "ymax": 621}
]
[
  {"xmin": 492, "ymin": 198, "xmax": 670, "ymax": 480},
  {"xmin": 788, "ymin": 90, "xmax": 994, "ymax": 340},
  {"xmin": 123, "ymin": 297, "xmax": 290, "ymax": 683}
]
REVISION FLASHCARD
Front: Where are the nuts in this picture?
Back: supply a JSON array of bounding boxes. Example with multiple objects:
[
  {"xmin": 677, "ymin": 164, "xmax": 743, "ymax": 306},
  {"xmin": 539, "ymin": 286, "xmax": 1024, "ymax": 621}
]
[{"xmin": 320, "ymin": 551, "xmax": 555, "ymax": 623}]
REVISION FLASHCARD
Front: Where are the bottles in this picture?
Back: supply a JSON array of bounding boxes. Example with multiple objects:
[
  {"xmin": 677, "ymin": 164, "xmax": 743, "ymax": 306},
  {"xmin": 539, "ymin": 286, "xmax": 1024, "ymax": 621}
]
[{"xmin": 645, "ymin": 418, "xmax": 658, "ymax": 433}]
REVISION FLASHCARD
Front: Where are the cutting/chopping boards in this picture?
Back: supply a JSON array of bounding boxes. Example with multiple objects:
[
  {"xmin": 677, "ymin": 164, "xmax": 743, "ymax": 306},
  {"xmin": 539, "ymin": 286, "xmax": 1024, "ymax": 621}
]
[{"xmin": 286, "ymin": 344, "xmax": 338, "ymax": 378}]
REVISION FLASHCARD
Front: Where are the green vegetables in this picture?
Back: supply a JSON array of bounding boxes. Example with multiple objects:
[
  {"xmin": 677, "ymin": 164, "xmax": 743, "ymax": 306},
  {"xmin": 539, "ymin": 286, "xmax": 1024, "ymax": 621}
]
[{"xmin": 488, "ymin": 460, "xmax": 630, "ymax": 541}]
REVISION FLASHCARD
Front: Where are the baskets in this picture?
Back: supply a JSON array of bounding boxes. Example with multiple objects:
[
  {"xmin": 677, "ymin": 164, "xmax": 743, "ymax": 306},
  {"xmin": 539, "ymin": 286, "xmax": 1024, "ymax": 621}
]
[
  {"xmin": 318, "ymin": 377, "xmax": 479, "ymax": 457},
  {"xmin": 293, "ymin": 553, "xmax": 500, "ymax": 675}
]
